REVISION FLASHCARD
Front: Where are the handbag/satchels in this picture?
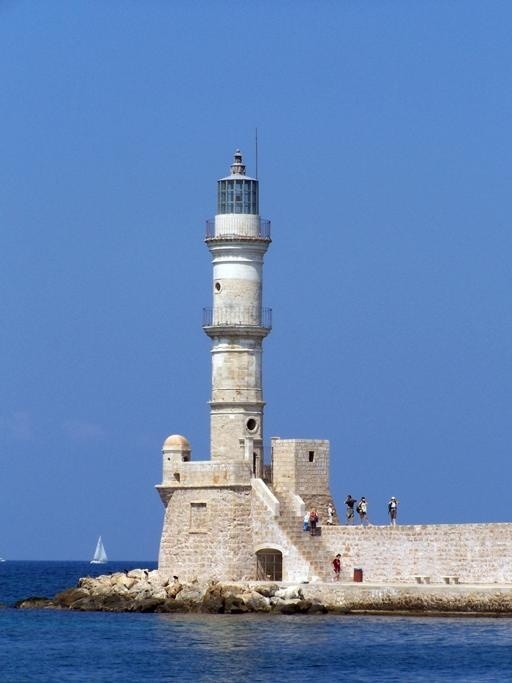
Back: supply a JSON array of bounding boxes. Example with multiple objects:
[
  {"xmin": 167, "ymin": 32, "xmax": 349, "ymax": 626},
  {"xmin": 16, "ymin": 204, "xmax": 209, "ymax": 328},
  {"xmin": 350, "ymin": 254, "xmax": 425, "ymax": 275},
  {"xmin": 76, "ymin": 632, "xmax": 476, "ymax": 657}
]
[
  {"xmin": 357, "ymin": 507, "xmax": 363, "ymax": 514},
  {"xmin": 315, "ymin": 516, "xmax": 318, "ymax": 522},
  {"xmin": 389, "ymin": 502, "xmax": 392, "ymax": 511}
]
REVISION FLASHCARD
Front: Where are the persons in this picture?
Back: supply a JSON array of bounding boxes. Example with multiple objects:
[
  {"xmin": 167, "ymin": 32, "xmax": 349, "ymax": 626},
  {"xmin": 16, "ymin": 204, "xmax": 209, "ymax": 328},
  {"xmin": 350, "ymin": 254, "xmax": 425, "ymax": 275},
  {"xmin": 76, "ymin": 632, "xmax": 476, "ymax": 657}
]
[
  {"xmin": 358, "ymin": 495, "xmax": 371, "ymax": 526},
  {"xmin": 387, "ymin": 495, "xmax": 399, "ymax": 526},
  {"xmin": 343, "ymin": 494, "xmax": 357, "ymax": 524},
  {"xmin": 331, "ymin": 553, "xmax": 342, "ymax": 582},
  {"xmin": 327, "ymin": 502, "xmax": 334, "ymax": 525},
  {"xmin": 310, "ymin": 506, "xmax": 319, "ymax": 535},
  {"xmin": 303, "ymin": 510, "xmax": 311, "ymax": 531}
]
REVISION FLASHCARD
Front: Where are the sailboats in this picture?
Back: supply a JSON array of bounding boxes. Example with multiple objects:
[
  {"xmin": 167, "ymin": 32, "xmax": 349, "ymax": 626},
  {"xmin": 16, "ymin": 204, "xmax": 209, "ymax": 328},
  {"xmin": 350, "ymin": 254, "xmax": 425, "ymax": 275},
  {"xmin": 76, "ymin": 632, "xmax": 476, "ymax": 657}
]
[{"xmin": 89, "ymin": 534, "xmax": 109, "ymax": 565}]
